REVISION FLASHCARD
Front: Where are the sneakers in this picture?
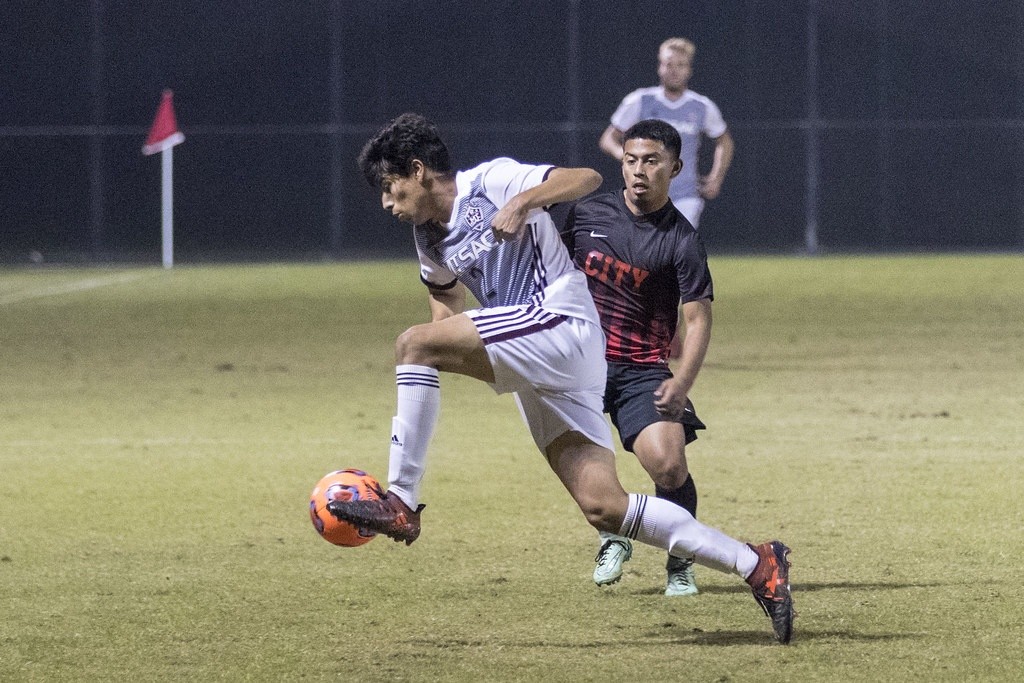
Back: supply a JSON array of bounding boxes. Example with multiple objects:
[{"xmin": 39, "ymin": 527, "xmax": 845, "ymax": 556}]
[
  {"xmin": 594, "ymin": 532, "xmax": 633, "ymax": 587},
  {"xmin": 745, "ymin": 543, "xmax": 795, "ymax": 644},
  {"xmin": 324, "ymin": 491, "xmax": 423, "ymax": 548},
  {"xmin": 664, "ymin": 551, "xmax": 700, "ymax": 598}
]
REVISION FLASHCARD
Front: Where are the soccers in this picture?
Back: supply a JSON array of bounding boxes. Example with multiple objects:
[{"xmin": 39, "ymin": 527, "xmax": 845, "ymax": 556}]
[{"xmin": 309, "ymin": 467, "xmax": 386, "ymax": 548}]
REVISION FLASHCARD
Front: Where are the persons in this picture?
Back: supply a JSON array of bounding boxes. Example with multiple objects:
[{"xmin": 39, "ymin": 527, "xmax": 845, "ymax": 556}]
[
  {"xmin": 596, "ymin": 38, "xmax": 737, "ymax": 359},
  {"xmin": 326, "ymin": 111, "xmax": 794, "ymax": 646},
  {"xmin": 545, "ymin": 116, "xmax": 716, "ymax": 599}
]
